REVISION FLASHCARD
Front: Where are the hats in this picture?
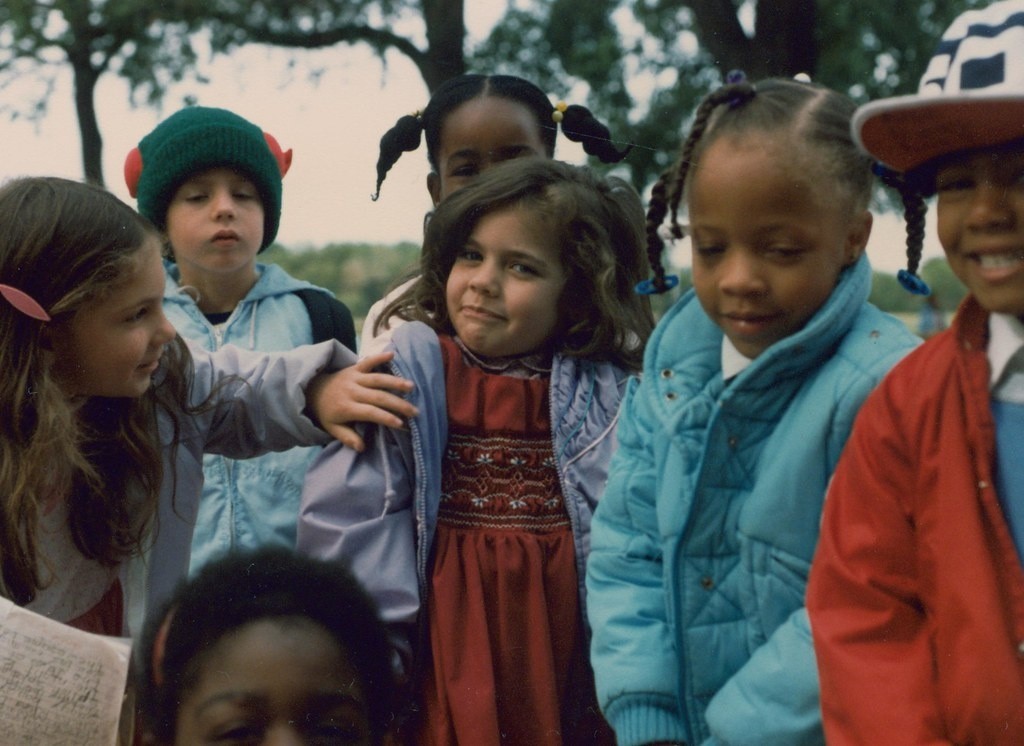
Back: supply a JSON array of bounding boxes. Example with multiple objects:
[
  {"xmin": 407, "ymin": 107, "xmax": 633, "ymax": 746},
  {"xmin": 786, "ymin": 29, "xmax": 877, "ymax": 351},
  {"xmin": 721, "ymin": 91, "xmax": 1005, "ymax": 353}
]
[
  {"xmin": 850, "ymin": 0, "xmax": 1024, "ymax": 171},
  {"xmin": 123, "ymin": 106, "xmax": 293, "ymax": 263}
]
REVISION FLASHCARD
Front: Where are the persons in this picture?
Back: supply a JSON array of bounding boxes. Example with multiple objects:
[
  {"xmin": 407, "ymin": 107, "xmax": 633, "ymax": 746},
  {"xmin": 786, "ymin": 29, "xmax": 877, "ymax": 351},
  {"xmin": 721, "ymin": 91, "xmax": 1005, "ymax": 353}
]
[
  {"xmin": 586, "ymin": 79, "xmax": 936, "ymax": 746},
  {"xmin": 122, "ymin": 108, "xmax": 359, "ymax": 637},
  {"xmin": 299, "ymin": 156, "xmax": 669, "ymax": 746},
  {"xmin": 360, "ymin": 74, "xmax": 629, "ymax": 378},
  {"xmin": 807, "ymin": 1, "xmax": 1024, "ymax": 745},
  {"xmin": 0, "ymin": 178, "xmax": 420, "ymax": 746},
  {"xmin": 136, "ymin": 546, "xmax": 394, "ymax": 746}
]
